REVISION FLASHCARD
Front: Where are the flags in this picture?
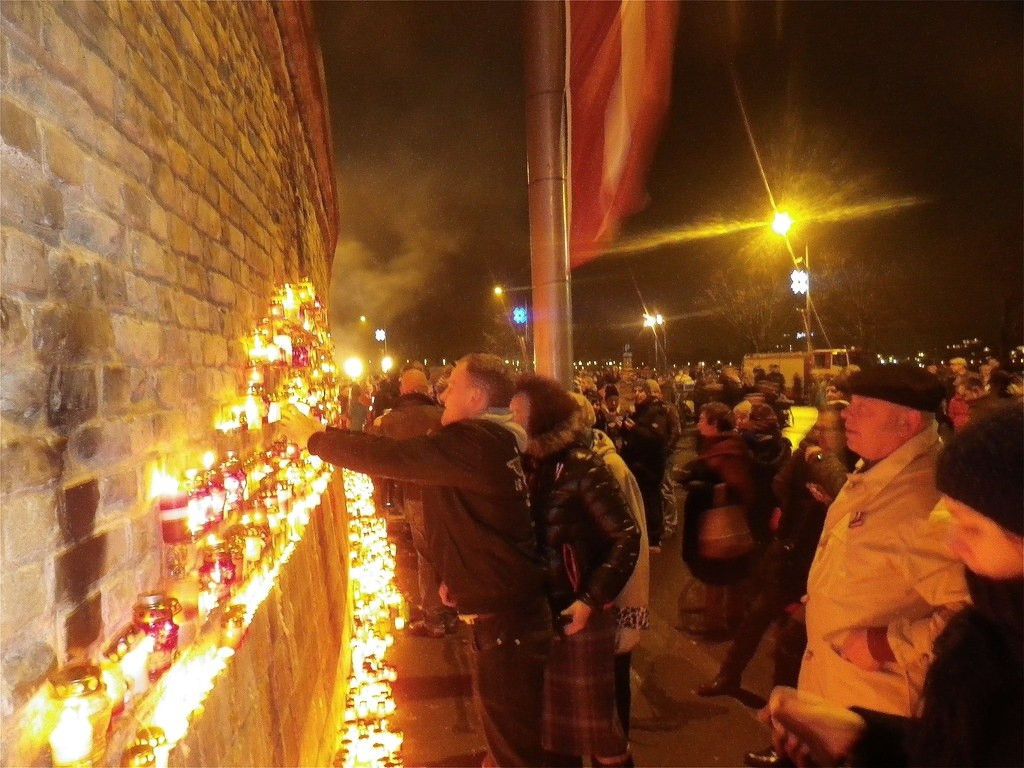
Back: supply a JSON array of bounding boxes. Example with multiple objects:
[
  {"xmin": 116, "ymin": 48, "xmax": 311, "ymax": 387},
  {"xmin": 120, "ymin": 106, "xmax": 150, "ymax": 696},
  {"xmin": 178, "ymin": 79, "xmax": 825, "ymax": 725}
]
[{"xmin": 566, "ymin": 0, "xmax": 682, "ymax": 273}]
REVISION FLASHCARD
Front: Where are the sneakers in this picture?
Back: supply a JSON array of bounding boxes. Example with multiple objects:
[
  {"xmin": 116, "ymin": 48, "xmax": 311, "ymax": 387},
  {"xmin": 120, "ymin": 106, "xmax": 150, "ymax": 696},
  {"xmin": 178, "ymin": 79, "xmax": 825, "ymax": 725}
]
[
  {"xmin": 442, "ymin": 612, "xmax": 459, "ymax": 632},
  {"xmin": 409, "ymin": 616, "xmax": 446, "ymax": 637}
]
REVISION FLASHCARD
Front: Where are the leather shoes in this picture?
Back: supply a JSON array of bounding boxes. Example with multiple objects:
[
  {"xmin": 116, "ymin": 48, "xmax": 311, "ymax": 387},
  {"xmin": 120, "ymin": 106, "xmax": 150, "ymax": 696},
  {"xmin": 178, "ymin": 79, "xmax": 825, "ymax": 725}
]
[
  {"xmin": 694, "ymin": 671, "xmax": 742, "ymax": 698},
  {"xmin": 746, "ymin": 746, "xmax": 785, "ymax": 765}
]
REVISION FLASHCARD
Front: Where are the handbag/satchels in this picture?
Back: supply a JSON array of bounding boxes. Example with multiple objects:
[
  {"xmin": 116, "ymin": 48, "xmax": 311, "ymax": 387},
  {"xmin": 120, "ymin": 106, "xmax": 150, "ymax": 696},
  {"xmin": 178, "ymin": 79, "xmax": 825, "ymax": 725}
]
[{"xmin": 698, "ymin": 486, "xmax": 754, "ymax": 559}]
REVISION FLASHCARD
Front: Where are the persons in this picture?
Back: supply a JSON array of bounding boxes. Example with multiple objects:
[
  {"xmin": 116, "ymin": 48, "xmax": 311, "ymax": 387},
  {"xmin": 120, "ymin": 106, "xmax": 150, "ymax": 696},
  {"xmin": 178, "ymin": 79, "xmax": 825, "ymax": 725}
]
[
  {"xmin": 499, "ymin": 374, "xmax": 646, "ymax": 768},
  {"xmin": 756, "ymin": 401, "xmax": 1022, "ymax": 767},
  {"xmin": 330, "ymin": 347, "xmax": 1023, "ymax": 636},
  {"xmin": 563, "ymin": 390, "xmax": 654, "ymax": 754},
  {"xmin": 795, "ymin": 359, "xmax": 975, "ymax": 719},
  {"xmin": 695, "ymin": 402, "xmax": 857, "ymax": 696},
  {"xmin": 276, "ymin": 354, "xmax": 553, "ymax": 768}
]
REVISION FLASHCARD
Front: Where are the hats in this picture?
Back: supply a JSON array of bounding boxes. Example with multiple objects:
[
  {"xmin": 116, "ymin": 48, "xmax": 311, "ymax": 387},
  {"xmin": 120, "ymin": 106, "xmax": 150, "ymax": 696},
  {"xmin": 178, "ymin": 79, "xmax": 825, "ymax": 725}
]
[
  {"xmin": 632, "ymin": 379, "xmax": 652, "ymax": 397},
  {"xmin": 842, "ymin": 362, "xmax": 946, "ymax": 412},
  {"xmin": 605, "ymin": 385, "xmax": 619, "ymax": 398},
  {"xmin": 936, "ymin": 396, "xmax": 1024, "ymax": 535}
]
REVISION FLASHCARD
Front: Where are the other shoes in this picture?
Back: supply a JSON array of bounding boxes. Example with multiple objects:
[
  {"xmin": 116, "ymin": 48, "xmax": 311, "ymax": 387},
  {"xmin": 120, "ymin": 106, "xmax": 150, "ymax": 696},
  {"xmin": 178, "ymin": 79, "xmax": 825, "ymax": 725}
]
[{"xmin": 674, "ymin": 621, "xmax": 725, "ymax": 641}]
